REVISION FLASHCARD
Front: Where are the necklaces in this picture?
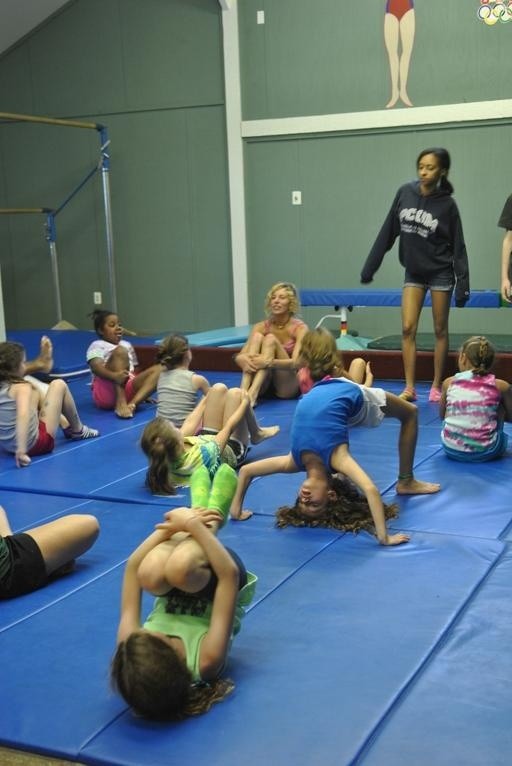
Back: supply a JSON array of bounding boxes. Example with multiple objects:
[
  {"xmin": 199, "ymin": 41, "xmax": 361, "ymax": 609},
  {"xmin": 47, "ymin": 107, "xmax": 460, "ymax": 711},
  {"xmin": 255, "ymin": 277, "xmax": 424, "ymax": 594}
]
[{"xmin": 274, "ymin": 316, "xmax": 292, "ymax": 329}]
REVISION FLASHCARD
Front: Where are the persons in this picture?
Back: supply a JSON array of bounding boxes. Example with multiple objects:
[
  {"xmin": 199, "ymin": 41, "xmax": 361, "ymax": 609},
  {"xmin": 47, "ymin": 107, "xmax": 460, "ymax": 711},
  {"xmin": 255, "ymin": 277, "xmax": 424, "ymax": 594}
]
[
  {"xmin": 360, "ymin": 148, "xmax": 471, "ymax": 405},
  {"xmin": 155, "ymin": 330, "xmax": 212, "ymax": 431},
  {"xmin": 0, "ymin": 342, "xmax": 101, "ymax": 468},
  {"xmin": 110, "ymin": 463, "xmax": 256, "ymax": 724},
  {"xmin": 296, "ymin": 326, "xmax": 373, "ymax": 404},
  {"xmin": 498, "ymin": 177, "xmax": 511, "ymax": 315},
  {"xmin": 439, "ymin": 334, "xmax": 511, "ymax": 463},
  {"xmin": 85, "ymin": 309, "xmax": 166, "ymax": 419},
  {"xmin": 138, "ymin": 382, "xmax": 280, "ymax": 497},
  {"xmin": 382, "ymin": 0, "xmax": 418, "ymax": 108},
  {"xmin": 25, "ymin": 334, "xmax": 54, "ymax": 377},
  {"xmin": 0, "ymin": 495, "xmax": 100, "ymax": 600},
  {"xmin": 232, "ymin": 281, "xmax": 309, "ymax": 408},
  {"xmin": 229, "ymin": 377, "xmax": 441, "ymax": 547}
]
